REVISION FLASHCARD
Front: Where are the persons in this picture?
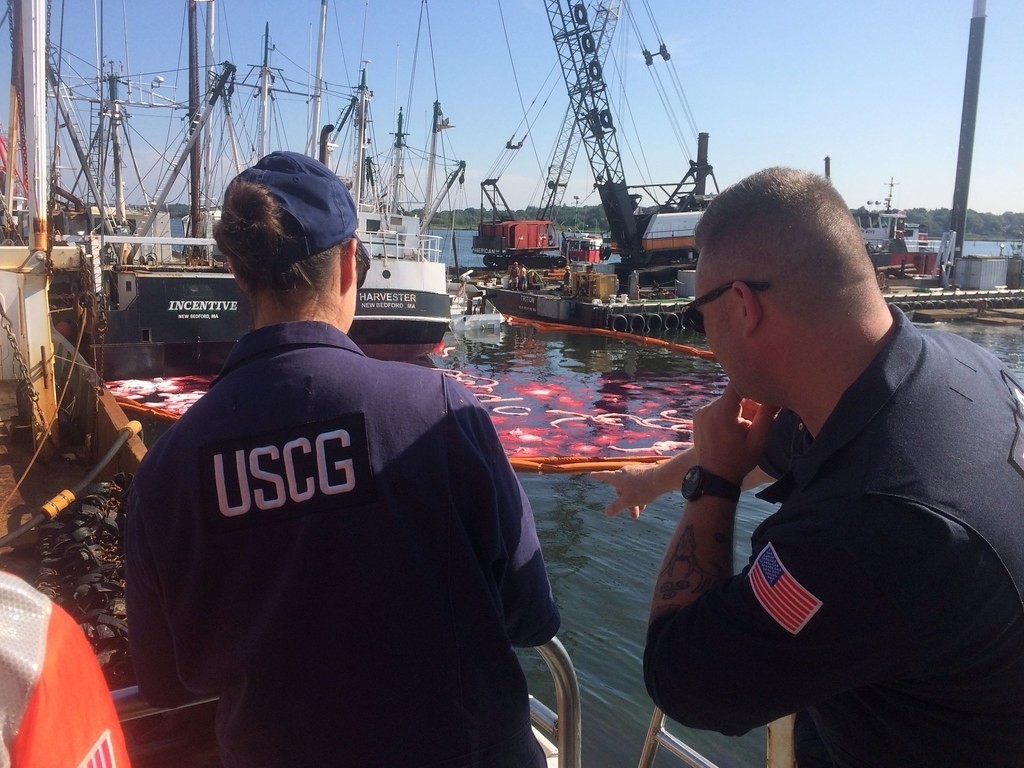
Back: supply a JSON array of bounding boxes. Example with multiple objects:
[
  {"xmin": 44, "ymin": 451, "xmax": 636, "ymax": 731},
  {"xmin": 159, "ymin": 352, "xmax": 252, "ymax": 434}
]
[
  {"xmin": 563, "ymin": 265, "xmax": 571, "ymax": 295},
  {"xmin": 520, "ymin": 264, "xmax": 528, "ymax": 291},
  {"xmin": 102, "ymin": 150, "xmax": 560, "ymax": 768},
  {"xmin": 511, "ymin": 262, "xmax": 519, "ymax": 289},
  {"xmin": 589, "ymin": 168, "xmax": 1024, "ymax": 768}
]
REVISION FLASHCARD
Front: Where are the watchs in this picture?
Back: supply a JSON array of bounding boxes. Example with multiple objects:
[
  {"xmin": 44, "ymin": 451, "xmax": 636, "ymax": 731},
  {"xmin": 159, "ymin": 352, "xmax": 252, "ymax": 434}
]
[{"xmin": 681, "ymin": 465, "xmax": 743, "ymax": 504}]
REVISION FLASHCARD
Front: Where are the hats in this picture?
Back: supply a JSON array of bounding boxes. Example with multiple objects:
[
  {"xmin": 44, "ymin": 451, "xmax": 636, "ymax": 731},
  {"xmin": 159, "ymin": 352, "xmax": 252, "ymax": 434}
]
[{"xmin": 226, "ymin": 153, "xmax": 356, "ymax": 270}]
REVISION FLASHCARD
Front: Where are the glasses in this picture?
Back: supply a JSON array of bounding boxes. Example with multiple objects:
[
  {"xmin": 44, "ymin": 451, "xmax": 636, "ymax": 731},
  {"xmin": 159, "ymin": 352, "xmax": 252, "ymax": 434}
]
[
  {"xmin": 682, "ymin": 279, "xmax": 770, "ymax": 336},
  {"xmin": 350, "ymin": 231, "xmax": 371, "ymax": 288}
]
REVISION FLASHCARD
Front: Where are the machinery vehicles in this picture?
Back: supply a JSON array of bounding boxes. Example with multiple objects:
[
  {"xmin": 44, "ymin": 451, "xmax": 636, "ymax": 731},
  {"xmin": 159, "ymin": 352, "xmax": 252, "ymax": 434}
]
[
  {"xmin": 473, "ymin": 0, "xmax": 625, "ymax": 271},
  {"xmin": 543, "ymin": 0, "xmax": 726, "ymax": 291}
]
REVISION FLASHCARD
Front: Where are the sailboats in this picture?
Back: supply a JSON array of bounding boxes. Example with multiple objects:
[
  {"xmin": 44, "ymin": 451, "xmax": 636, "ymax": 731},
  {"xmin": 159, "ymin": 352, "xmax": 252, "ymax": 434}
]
[{"xmin": 1, "ymin": 2, "xmax": 942, "ymax": 376}]
